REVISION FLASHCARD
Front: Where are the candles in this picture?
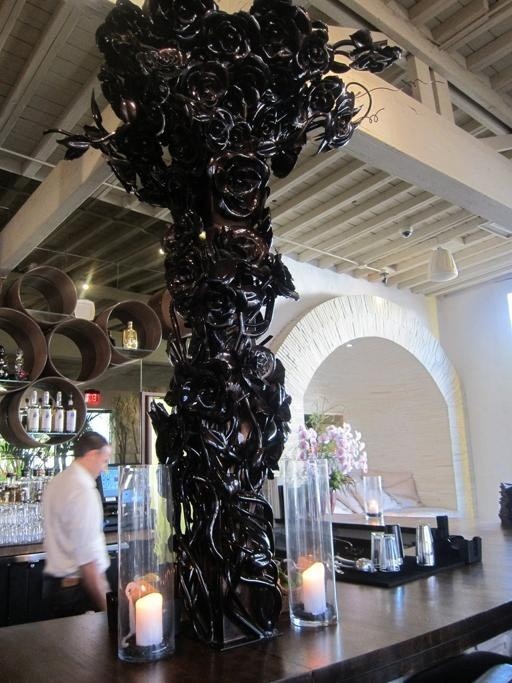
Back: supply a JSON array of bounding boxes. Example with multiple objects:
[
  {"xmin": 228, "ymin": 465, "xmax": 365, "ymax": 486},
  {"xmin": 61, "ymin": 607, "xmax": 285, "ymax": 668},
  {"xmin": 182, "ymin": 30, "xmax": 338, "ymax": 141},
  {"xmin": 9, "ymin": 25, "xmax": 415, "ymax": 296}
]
[
  {"xmin": 300, "ymin": 562, "xmax": 329, "ymax": 616},
  {"xmin": 368, "ymin": 497, "xmax": 379, "ymax": 517},
  {"xmin": 134, "ymin": 592, "xmax": 165, "ymax": 648}
]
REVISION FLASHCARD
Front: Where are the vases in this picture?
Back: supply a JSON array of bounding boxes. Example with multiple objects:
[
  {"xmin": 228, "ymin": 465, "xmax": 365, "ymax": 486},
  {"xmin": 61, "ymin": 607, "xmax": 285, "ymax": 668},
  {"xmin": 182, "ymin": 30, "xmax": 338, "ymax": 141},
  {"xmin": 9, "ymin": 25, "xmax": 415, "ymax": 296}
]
[{"xmin": 314, "ymin": 491, "xmax": 338, "ymax": 515}]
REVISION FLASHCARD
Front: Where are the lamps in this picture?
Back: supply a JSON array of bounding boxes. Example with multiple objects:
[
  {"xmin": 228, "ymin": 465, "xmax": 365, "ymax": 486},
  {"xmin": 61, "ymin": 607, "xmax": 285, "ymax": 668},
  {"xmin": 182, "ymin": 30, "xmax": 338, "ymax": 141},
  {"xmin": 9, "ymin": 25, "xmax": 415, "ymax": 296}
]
[{"xmin": 425, "ymin": 247, "xmax": 459, "ymax": 285}]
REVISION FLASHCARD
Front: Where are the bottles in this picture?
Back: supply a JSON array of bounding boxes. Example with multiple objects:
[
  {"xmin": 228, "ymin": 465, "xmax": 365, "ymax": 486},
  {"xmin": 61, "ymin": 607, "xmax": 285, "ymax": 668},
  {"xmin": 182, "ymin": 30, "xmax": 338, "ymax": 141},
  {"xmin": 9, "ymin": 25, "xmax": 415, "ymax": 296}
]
[
  {"xmin": 14, "ymin": 347, "xmax": 25, "ymax": 378},
  {"xmin": 22, "ymin": 391, "xmax": 78, "ymax": 433},
  {"xmin": 385, "ymin": 523, "xmax": 405, "ymax": 560},
  {"xmin": 1, "ymin": 346, "xmax": 9, "ymax": 378},
  {"xmin": 108, "ymin": 331, "xmax": 116, "ymax": 346},
  {"xmin": 415, "ymin": 522, "xmax": 437, "ymax": 566},
  {"xmin": 121, "ymin": 321, "xmax": 138, "ymax": 349},
  {"xmin": 0, "ymin": 468, "xmax": 54, "ymax": 502}
]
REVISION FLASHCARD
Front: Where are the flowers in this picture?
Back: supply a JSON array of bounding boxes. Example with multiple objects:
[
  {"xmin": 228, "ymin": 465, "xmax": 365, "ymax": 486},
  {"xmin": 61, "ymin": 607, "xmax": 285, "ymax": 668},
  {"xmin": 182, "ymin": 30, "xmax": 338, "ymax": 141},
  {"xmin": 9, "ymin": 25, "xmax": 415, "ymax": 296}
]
[{"xmin": 278, "ymin": 392, "xmax": 371, "ymax": 491}]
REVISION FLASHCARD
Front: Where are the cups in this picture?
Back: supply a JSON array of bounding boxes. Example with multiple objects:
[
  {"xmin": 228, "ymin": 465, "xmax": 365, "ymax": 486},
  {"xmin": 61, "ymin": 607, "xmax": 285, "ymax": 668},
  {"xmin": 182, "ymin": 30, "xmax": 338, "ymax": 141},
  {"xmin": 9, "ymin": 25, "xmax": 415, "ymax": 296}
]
[
  {"xmin": 0, "ymin": 502, "xmax": 47, "ymax": 544},
  {"xmin": 380, "ymin": 536, "xmax": 401, "ymax": 572},
  {"xmin": 362, "ymin": 476, "xmax": 384, "ymax": 516},
  {"xmin": 105, "ymin": 590, "xmax": 129, "ymax": 632},
  {"xmin": 370, "ymin": 531, "xmax": 384, "ymax": 569}
]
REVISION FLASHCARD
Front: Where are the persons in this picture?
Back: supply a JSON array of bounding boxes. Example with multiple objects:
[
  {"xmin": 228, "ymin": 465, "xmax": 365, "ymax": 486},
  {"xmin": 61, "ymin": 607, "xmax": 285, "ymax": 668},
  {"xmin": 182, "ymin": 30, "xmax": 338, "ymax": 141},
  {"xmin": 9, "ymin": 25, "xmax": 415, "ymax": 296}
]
[{"xmin": 42, "ymin": 430, "xmax": 112, "ymax": 615}]
